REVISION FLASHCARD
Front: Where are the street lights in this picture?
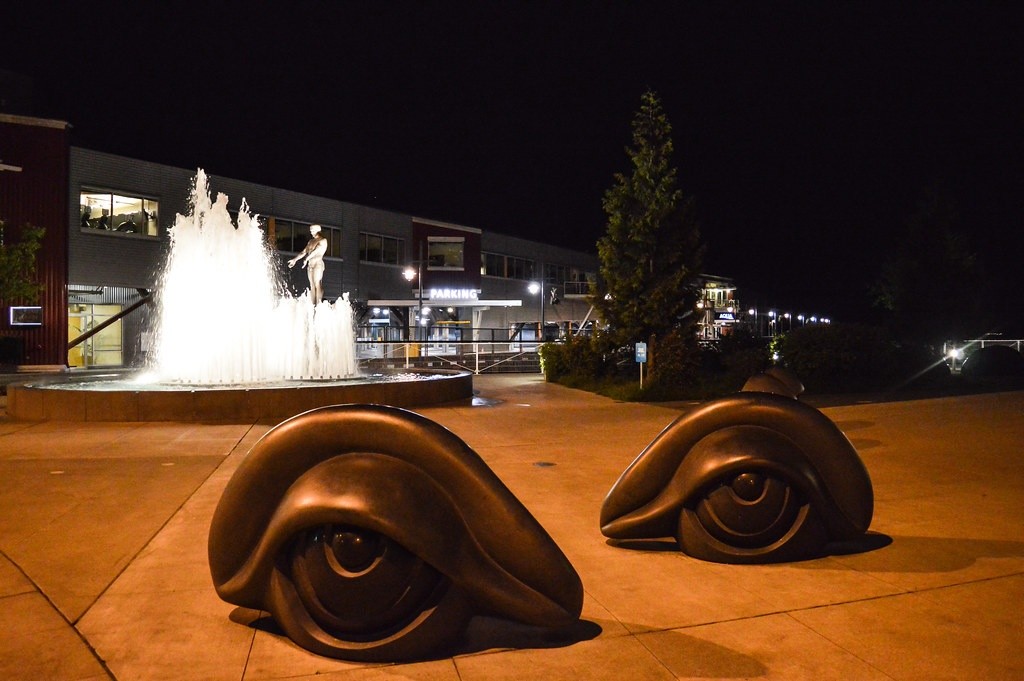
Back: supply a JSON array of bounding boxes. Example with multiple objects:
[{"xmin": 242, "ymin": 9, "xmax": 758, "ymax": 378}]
[
  {"xmin": 769, "ymin": 308, "xmax": 777, "ymax": 337},
  {"xmin": 527, "ymin": 263, "xmax": 546, "ymax": 342},
  {"xmin": 783, "ymin": 312, "xmax": 791, "ymax": 331},
  {"xmin": 798, "ymin": 313, "xmax": 805, "ymax": 327},
  {"xmin": 748, "ymin": 305, "xmax": 758, "ymax": 333},
  {"xmin": 727, "ymin": 300, "xmax": 738, "ymax": 323},
  {"xmin": 401, "ymin": 260, "xmax": 423, "ymax": 361}
]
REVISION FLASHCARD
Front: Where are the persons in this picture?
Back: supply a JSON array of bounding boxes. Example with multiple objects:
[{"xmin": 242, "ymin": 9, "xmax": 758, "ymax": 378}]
[
  {"xmin": 286, "ymin": 224, "xmax": 327, "ymax": 303},
  {"xmin": 82, "ymin": 206, "xmax": 139, "ymax": 233}
]
